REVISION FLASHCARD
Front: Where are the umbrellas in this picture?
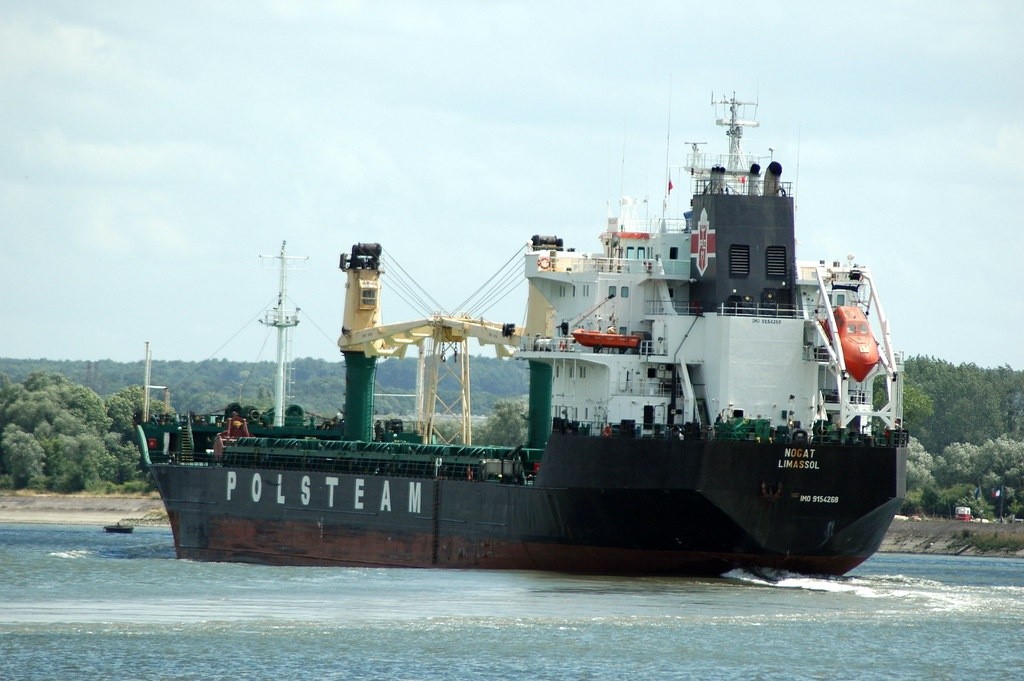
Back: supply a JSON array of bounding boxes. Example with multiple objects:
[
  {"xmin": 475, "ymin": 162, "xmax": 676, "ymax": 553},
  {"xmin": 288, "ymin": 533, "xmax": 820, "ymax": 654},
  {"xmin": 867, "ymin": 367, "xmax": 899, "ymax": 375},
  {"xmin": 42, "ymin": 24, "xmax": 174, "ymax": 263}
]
[{"xmin": 814, "ymin": 390, "xmax": 828, "ymax": 441}]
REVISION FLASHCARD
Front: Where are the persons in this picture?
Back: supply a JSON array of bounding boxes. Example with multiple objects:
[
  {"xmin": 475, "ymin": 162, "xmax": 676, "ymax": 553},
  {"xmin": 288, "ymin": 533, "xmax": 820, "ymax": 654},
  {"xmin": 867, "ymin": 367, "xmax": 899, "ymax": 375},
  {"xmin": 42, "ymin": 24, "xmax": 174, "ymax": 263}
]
[
  {"xmin": 521, "ymin": 333, "xmax": 577, "ymax": 352},
  {"xmin": 788, "ymin": 418, "xmax": 794, "ymax": 438},
  {"xmin": 756, "ymin": 414, "xmax": 762, "ymax": 419},
  {"xmin": 884, "ymin": 420, "xmax": 904, "ymax": 447},
  {"xmin": 604, "ymin": 422, "xmax": 613, "ymax": 438},
  {"xmin": 607, "ymin": 325, "xmax": 617, "ymax": 334},
  {"xmin": 716, "ymin": 413, "xmax": 732, "ymax": 423}
]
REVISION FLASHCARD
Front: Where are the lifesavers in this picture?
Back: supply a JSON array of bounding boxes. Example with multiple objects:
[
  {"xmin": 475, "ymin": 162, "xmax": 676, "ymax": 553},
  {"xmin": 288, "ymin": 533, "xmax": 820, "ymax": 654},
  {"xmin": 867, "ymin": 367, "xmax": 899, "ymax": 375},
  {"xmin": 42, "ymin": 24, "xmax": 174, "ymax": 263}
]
[
  {"xmin": 537, "ymin": 256, "xmax": 552, "ymax": 270},
  {"xmin": 791, "ymin": 429, "xmax": 809, "ymax": 444},
  {"xmin": 602, "ymin": 426, "xmax": 613, "ymax": 437}
]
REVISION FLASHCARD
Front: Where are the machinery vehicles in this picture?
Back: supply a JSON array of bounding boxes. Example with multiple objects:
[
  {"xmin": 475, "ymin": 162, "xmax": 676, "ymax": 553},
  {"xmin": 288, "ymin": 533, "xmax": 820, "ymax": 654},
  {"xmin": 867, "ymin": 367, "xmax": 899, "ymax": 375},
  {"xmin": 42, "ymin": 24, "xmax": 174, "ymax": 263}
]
[{"xmin": 552, "ymin": 293, "xmax": 615, "ymax": 352}]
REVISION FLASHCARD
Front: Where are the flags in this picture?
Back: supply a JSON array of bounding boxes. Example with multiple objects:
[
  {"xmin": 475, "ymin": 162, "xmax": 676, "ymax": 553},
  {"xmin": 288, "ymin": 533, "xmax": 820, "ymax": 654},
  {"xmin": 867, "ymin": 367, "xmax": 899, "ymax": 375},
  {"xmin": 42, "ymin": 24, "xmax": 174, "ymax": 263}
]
[
  {"xmin": 988, "ymin": 485, "xmax": 1003, "ymax": 498},
  {"xmin": 974, "ymin": 483, "xmax": 981, "ymax": 500}
]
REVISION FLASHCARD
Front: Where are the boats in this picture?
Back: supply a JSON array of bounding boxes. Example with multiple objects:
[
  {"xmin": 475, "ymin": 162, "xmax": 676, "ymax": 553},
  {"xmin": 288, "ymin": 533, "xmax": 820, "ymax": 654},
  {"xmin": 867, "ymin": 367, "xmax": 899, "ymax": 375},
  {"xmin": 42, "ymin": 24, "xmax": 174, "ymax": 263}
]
[
  {"xmin": 135, "ymin": 90, "xmax": 909, "ymax": 579},
  {"xmin": 105, "ymin": 525, "xmax": 133, "ymax": 533},
  {"xmin": 571, "ymin": 328, "xmax": 642, "ymax": 347}
]
